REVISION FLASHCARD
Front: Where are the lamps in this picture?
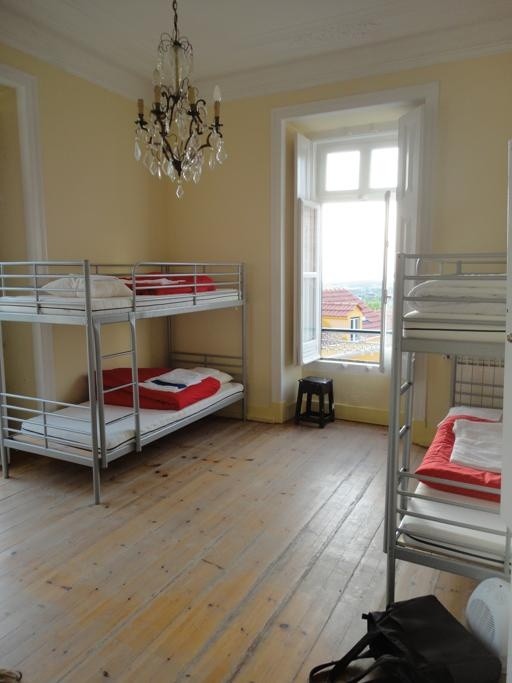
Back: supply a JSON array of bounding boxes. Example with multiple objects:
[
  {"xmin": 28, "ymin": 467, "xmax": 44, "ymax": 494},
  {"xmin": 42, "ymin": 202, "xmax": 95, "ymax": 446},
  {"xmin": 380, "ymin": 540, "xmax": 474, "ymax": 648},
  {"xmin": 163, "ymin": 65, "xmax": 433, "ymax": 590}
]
[{"xmin": 132, "ymin": 0, "xmax": 228, "ymax": 199}]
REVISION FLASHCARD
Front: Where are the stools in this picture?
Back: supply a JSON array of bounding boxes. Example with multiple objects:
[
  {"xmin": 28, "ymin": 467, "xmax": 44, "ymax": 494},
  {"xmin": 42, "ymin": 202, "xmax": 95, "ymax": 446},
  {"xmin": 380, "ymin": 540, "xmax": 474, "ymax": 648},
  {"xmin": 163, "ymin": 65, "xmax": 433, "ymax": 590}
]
[{"xmin": 293, "ymin": 377, "xmax": 336, "ymax": 429}]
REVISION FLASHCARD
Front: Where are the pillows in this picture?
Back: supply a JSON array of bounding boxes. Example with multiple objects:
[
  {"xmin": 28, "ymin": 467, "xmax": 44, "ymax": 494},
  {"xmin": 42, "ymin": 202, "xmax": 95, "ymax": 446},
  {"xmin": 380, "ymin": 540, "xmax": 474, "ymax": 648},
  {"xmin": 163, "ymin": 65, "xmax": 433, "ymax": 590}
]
[
  {"xmin": 406, "ymin": 279, "xmax": 507, "ymax": 317},
  {"xmin": 39, "ymin": 274, "xmax": 130, "ymax": 297}
]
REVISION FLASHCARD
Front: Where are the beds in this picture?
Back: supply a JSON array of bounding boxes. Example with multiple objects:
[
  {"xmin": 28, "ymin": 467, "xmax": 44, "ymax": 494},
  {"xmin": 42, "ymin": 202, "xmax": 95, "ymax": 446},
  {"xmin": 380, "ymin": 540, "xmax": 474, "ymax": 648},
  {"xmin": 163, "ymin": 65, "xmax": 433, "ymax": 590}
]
[
  {"xmin": 0, "ymin": 258, "xmax": 249, "ymax": 506},
  {"xmin": 384, "ymin": 252, "xmax": 512, "ymax": 613}
]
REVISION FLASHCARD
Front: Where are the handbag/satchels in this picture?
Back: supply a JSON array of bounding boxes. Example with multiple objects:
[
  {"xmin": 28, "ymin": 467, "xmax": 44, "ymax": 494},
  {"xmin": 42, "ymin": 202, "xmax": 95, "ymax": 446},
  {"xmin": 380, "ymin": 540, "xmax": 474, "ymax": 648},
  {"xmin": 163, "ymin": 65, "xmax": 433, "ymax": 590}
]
[{"xmin": 310, "ymin": 595, "xmax": 502, "ymax": 682}]
